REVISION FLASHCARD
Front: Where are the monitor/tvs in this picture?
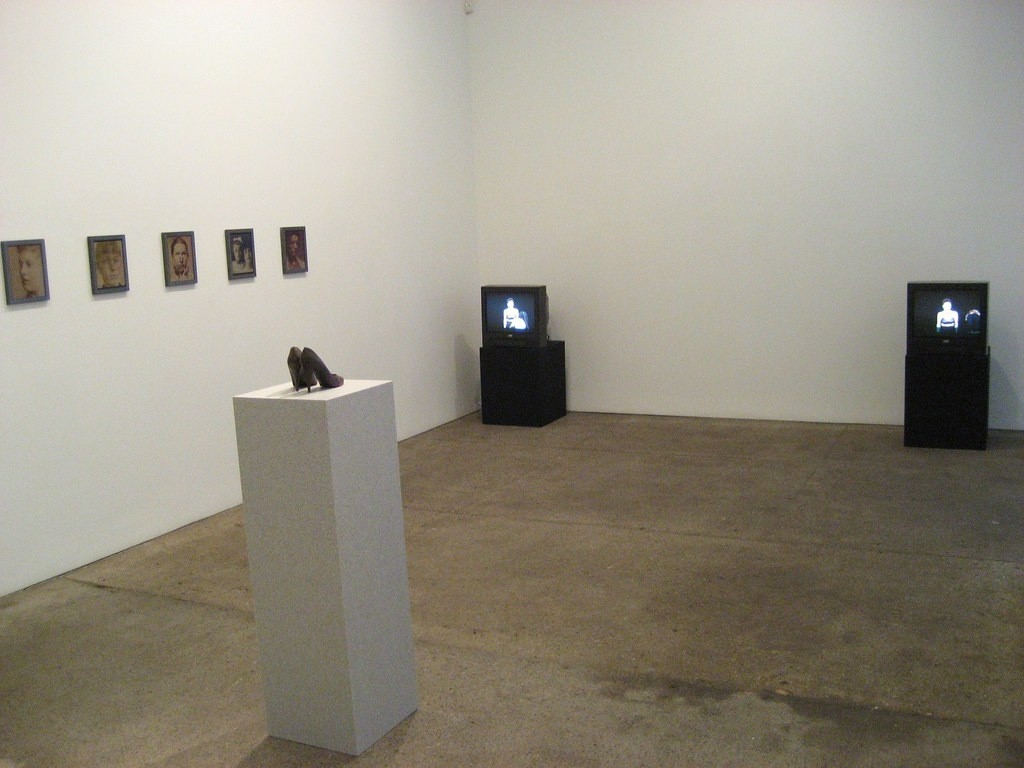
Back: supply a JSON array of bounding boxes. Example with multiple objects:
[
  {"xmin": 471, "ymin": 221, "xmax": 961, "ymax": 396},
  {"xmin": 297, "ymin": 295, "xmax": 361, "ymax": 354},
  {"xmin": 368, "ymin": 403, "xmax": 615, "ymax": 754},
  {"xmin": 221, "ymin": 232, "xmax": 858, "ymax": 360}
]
[
  {"xmin": 908, "ymin": 280, "xmax": 990, "ymax": 356},
  {"xmin": 481, "ymin": 285, "xmax": 549, "ymax": 348}
]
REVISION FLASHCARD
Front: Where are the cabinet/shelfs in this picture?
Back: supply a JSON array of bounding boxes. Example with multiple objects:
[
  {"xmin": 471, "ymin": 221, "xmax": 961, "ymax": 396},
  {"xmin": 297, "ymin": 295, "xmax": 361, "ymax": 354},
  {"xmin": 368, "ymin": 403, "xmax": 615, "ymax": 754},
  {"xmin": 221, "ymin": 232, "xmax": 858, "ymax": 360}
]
[
  {"xmin": 904, "ymin": 345, "xmax": 991, "ymax": 451},
  {"xmin": 480, "ymin": 341, "xmax": 566, "ymax": 427}
]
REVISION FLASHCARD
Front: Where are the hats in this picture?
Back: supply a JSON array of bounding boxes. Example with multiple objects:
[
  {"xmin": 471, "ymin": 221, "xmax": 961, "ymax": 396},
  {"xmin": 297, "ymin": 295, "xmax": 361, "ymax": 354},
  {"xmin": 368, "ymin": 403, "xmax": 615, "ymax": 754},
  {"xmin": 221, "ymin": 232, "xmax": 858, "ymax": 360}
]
[
  {"xmin": 231, "ymin": 236, "xmax": 243, "ymax": 245},
  {"xmin": 95, "ymin": 241, "xmax": 122, "ymax": 259}
]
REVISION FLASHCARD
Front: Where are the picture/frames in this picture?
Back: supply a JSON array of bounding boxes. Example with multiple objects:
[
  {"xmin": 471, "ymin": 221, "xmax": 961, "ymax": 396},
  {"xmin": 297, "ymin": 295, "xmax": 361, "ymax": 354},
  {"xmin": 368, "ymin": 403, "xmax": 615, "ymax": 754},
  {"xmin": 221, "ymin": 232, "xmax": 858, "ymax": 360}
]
[
  {"xmin": 86, "ymin": 234, "xmax": 130, "ymax": 295},
  {"xmin": 225, "ymin": 229, "xmax": 257, "ymax": 279},
  {"xmin": 0, "ymin": 239, "xmax": 51, "ymax": 306},
  {"xmin": 161, "ymin": 230, "xmax": 198, "ymax": 286},
  {"xmin": 279, "ymin": 226, "xmax": 308, "ymax": 274}
]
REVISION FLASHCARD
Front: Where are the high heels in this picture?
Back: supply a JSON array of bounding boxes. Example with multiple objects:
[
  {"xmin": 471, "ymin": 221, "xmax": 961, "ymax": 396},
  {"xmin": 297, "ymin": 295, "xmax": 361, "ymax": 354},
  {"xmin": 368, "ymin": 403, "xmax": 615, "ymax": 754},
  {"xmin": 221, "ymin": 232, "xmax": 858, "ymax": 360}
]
[{"xmin": 288, "ymin": 347, "xmax": 344, "ymax": 393}]
